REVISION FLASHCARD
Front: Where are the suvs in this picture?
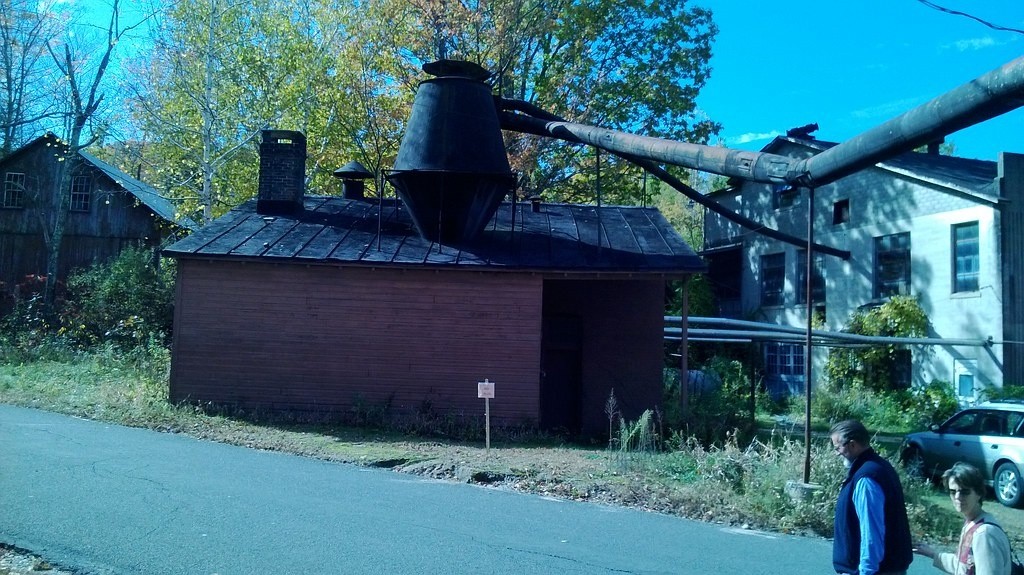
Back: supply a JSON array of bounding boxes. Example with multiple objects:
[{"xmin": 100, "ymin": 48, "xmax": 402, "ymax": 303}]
[{"xmin": 895, "ymin": 396, "xmax": 1024, "ymax": 508}]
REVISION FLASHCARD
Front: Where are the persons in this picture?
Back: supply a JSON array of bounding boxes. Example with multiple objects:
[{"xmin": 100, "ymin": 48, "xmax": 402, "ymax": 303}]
[
  {"xmin": 827, "ymin": 419, "xmax": 914, "ymax": 575},
  {"xmin": 909, "ymin": 461, "xmax": 1014, "ymax": 574}
]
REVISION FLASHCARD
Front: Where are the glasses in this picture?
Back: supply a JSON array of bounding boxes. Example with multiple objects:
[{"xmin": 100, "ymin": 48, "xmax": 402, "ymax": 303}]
[{"xmin": 946, "ymin": 487, "xmax": 974, "ymax": 497}]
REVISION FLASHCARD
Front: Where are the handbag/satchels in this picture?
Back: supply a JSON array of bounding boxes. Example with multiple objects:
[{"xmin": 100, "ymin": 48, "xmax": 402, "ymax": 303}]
[{"xmin": 968, "ymin": 563, "xmax": 1024, "ymax": 575}]
